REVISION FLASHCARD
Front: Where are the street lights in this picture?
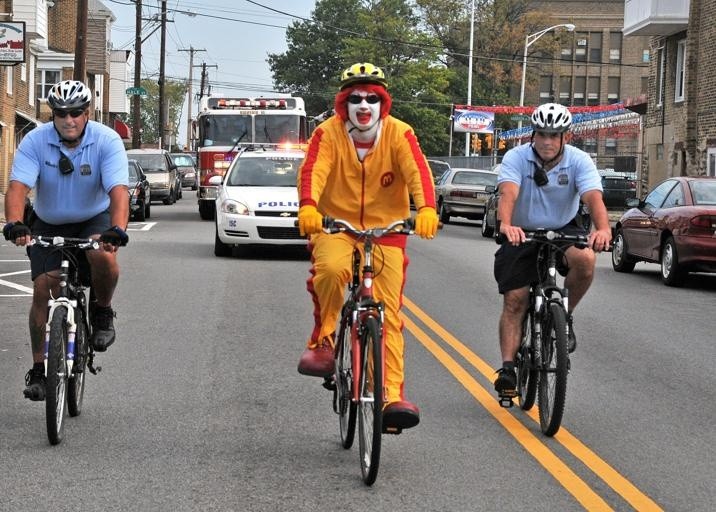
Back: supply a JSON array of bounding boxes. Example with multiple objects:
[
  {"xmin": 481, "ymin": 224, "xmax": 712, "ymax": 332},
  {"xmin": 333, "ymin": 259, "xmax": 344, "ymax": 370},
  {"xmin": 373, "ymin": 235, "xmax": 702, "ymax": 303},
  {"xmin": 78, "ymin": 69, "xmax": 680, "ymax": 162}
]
[
  {"xmin": 518, "ymin": 23, "xmax": 575, "ymax": 146},
  {"xmin": 152, "ymin": 8, "xmax": 196, "ymax": 146}
]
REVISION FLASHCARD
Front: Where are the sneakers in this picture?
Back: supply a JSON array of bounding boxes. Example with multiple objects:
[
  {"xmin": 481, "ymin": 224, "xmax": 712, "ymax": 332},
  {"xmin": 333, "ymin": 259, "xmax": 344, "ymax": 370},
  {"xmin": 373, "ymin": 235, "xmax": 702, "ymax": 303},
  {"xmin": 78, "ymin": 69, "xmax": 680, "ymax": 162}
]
[
  {"xmin": 494, "ymin": 368, "xmax": 517, "ymax": 392},
  {"xmin": 567, "ymin": 316, "xmax": 576, "ymax": 352},
  {"xmin": 23, "ymin": 369, "xmax": 46, "ymax": 398},
  {"xmin": 92, "ymin": 308, "xmax": 116, "ymax": 350}
]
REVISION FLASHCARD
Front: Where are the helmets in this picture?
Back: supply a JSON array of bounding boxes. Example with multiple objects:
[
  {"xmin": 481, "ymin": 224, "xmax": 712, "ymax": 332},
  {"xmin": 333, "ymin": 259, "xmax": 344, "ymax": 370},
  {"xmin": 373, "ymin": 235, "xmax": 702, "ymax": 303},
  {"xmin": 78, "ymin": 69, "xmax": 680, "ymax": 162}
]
[
  {"xmin": 46, "ymin": 80, "xmax": 92, "ymax": 110},
  {"xmin": 338, "ymin": 62, "xmax": 388, "ymax": 91},
  {"xmin": 531, "ymin": 103, "xmax": 572, "ymax": 131}
]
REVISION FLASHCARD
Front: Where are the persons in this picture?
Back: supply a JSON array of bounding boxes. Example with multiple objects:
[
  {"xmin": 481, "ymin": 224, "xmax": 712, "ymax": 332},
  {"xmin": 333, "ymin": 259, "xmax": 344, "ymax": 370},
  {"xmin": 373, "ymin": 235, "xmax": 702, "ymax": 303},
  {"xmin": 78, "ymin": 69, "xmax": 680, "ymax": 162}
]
[
  {"xmin": 294, "ymin": 61, "xmax": 443, "ymax": 486},
  {"xmin": 279, "ymin": 121, "xmax": 297, "ymax": 144},
  {"xmin": 2, "ymin": 79, "xmax": 131, "ymax": 446},
  {"xmin": 494, "ymin": 103, "xmax": 613, "ymax": 437}
]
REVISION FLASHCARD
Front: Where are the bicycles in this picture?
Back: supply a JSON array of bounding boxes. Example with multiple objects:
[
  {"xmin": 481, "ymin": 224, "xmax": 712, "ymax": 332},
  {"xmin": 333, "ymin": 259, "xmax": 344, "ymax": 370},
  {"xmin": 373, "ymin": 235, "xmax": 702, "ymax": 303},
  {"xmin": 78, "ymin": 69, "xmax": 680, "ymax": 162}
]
[
  {"xmin": 1, "ymin": 229, "xmax": 121, "ymax": 444},
  {"xmin": 294, "ymin": 214, "xmax": 446, "ymax": 487},
  {"xmin": 493, "ymin": 230, "xmax": 615, "ymax": 437}
]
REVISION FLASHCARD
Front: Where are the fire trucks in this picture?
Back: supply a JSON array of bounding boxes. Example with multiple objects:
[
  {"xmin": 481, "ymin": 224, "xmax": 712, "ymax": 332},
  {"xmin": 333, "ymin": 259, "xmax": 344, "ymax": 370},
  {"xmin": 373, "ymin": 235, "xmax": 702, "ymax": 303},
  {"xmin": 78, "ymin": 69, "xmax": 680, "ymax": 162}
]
[{"xmin": 192, "ymin": 97, "xmax": 306, "ymax": 219}]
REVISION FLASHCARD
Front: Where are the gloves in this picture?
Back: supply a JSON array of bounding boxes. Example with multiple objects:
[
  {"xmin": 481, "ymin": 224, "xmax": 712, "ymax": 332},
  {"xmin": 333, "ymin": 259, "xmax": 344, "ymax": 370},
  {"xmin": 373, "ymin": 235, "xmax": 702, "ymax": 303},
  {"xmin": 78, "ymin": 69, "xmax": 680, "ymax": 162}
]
[
  {"xmin": 102, "ymin": 226, "xmax": 128, "ymax": 246},
  {"xmin": 415, "ymin": 207, "xmax": 439, "ymax": 239},
  {"xmin": 298, "ymin": 205, "xmax": 324, "ymax": 236},
  {"xmin": 2, "ymin": 221, "xmax": 31, "ymax": 242}
]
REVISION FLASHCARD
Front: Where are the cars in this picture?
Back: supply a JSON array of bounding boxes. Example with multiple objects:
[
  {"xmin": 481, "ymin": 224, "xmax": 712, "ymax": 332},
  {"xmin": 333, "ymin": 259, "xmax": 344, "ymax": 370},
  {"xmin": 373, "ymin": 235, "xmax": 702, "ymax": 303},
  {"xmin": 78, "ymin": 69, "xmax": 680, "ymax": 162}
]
[
  {"xmin": 435, "ymin": 168, "xmax": 497, "ymax": 223},
  {"xmin": 411, "ymin": 160, "xmax": 451, "ymax": 209},
  {"xmin": 169, "ymin": 152, "xmax": 197, "ymax": 190},
  {"xmin": 481, "ymin": 183, "xmax": 498, "ymax": 236},
  {"xmin": 209, "ymin": 149, "xmax": 306, "ymax": 258},
  {"xmin": 127, "ymin": 160, "xmax": 150, "ymax": 220},
  {"xmin": 597, "ymin": 169, "xmax": 636, "ymax": 206},
  {"xmin": 612, "ymin": 176, "xmax": 716, "ymax": 284},
  {"xmin": 126, "ymin": 149, "xmax": 181, "ymax": 205}
]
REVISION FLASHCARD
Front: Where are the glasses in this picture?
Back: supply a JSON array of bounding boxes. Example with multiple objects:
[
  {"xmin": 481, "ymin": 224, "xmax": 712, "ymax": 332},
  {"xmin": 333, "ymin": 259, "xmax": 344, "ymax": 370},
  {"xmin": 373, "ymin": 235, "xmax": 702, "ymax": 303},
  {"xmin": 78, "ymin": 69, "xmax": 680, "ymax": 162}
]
[
  {"xmin": 346, "ymin": 95, "xmax": 381, "ymax": 103},
  {"xmin": 54, "ymin": 110, "xmax": 83, "ymax": 117}
]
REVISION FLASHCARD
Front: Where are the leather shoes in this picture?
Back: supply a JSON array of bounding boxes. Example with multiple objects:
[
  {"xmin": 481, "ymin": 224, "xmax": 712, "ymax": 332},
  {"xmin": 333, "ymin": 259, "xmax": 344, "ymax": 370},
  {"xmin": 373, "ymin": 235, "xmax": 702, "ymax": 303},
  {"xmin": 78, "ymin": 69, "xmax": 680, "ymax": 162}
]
[
  {"xmin": 383, "ymin": 401, "xmax": 419, "ymax": 428},
  {"xmin": 298, "ymin": 332, "xmax": 336, "ymax": 376}
]
